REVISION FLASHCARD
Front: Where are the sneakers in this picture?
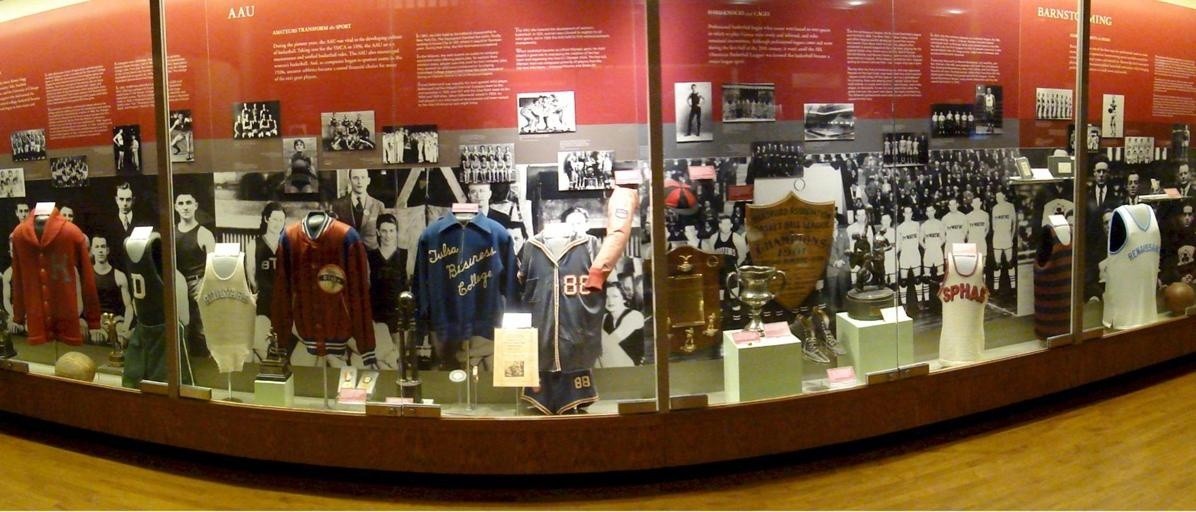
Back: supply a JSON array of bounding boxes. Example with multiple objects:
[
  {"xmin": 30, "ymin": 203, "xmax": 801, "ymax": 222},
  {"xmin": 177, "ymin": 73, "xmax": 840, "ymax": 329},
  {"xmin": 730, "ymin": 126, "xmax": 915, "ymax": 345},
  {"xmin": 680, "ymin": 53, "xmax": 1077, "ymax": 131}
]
[
  {"xmin": 989, "ymin": 291, "xmax": 1000, "ymax": 300},
  {"xmin": 186, "ymin": 153, "xmax": 191, "ymax": 160},
  {"xmin": 1010, "ymin": 288, "xmax": 1017, "ymax": 299},
  {"xmin": 174, "ymin": 149, "xmax": 181, "ymax": 154},
  {"xmin": 791, "ymin": 317, "xmax": 831, "ymax": 365},
  {"xmin": 901, "ymin": 300, "xmax": 931, "ymax": 317},
  {"xmin": 810, "ymin": 307, "xmax": 848, "ymax": 356}
]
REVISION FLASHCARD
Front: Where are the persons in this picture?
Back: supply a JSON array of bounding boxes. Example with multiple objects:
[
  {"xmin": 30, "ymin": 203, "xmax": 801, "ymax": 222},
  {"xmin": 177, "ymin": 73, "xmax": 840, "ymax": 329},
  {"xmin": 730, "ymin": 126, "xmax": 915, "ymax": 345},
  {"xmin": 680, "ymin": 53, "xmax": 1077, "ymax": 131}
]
[
  {"xmin": 938, "ymin": 243, "xmax": 989, "ymax": 360},
  {"xmin": 1, "ymin": 127, "xmax": 147, "ymax": 347},
  {"xmin": 196, "ymin": 210, "xmax": 607, "ymax": 413},
  {"xmin": 1087, "ymin": 92, "xmax": 1191, "ymax": 335},
  {"xmin": 172, "ymin": 96, "xmax": 651, "ymax": 372},
  {"xmin": 668, "ymin": 80, "xmax": 1032, "ymax": 311},
  {"xmin": 1033, "ymin": 214, "xmax": 1072, "ymax": 339},
  {"xmin": 9, "ymin": 203, "xmax": 196, "ymax": 389}
]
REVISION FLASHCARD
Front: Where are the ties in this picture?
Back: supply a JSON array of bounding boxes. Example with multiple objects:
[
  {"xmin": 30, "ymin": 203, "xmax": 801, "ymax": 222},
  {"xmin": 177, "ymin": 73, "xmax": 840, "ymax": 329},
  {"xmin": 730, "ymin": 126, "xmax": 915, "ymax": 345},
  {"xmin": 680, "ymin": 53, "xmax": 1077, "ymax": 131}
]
[
  {"xmin": 356, "ymin": 196, "xmax": 362, "ymax": 209},
  {"xmin": 1132, "ymin": 196, "xmax": 1135, "ymax": 205},
  {"xmin": 1098, "ymin": 184, "xmax": 1105, "ymax": 207},
  {"xmin": 1183, "ymin": 188, "xmax": 1185, "ymax": 196}
]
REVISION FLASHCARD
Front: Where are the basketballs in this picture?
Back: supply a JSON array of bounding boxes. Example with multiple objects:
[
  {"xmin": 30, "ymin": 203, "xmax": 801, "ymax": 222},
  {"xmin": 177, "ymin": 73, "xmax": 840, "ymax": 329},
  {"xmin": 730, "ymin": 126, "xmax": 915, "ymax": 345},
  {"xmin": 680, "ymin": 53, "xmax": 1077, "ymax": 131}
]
[{"xmin": 55, "ymin": 351, "xmax": 96, "ymax": 383}]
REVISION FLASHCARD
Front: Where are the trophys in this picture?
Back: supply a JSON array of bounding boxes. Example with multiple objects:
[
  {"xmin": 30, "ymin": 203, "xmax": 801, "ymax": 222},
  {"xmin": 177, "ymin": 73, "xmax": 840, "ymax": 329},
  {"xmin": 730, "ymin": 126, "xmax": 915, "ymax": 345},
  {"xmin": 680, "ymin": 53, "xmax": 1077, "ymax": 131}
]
[{"xmin": 727, "ymin": 266, "xmax": 787, "ymax": 337}]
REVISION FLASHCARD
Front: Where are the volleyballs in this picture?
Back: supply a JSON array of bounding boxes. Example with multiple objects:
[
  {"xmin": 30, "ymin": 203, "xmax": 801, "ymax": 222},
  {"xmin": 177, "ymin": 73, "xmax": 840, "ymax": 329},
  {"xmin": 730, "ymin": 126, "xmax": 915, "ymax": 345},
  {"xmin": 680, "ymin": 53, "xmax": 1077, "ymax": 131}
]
[{"xmin": 1164, "ymin": 282, "xmax": 1196, "ymax": 313}]
